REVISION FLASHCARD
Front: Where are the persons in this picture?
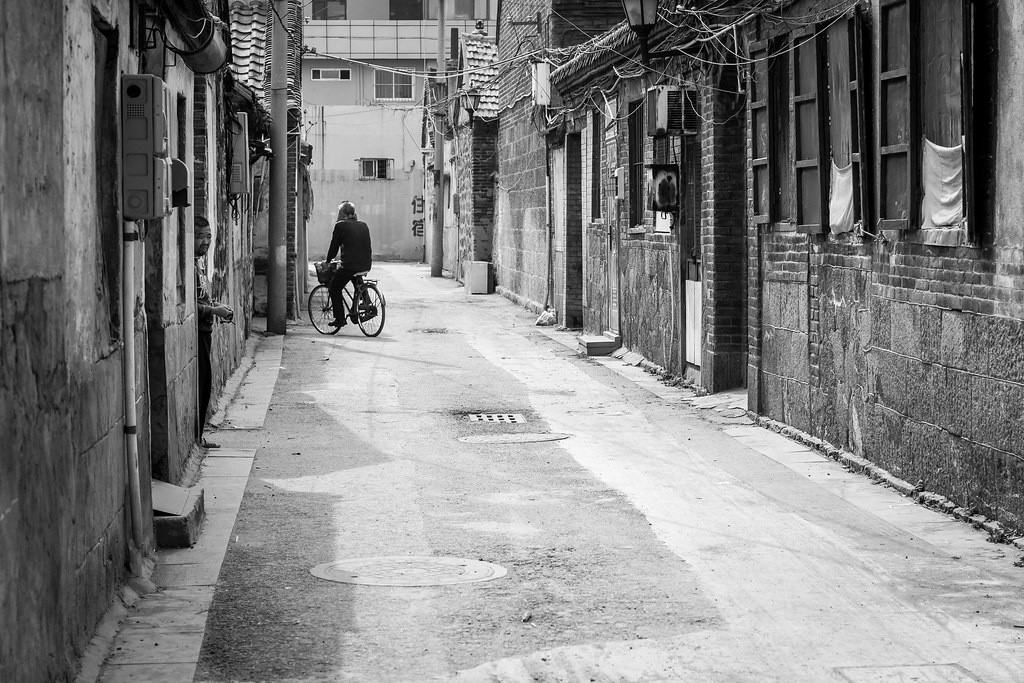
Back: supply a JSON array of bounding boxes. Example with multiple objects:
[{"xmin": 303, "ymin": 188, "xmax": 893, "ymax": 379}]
[
  {"xmin": 194, "ymin": 216, "xmax": 233, "ymax": 448},
  {"xmin": 326, "ymin": 202, "xmax": 378, "ymax": 326}
]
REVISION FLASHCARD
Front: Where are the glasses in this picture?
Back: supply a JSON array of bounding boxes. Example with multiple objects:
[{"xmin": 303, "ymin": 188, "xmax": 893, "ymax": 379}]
[{"xmin": 342, "ymin": 200, "xmax": 349, "ymax": 203}]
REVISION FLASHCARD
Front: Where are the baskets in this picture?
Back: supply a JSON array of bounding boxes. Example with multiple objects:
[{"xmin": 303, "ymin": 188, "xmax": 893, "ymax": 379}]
[{"xmin": 314, "ymin": 262, "xmax": 337, "ymax": 283}]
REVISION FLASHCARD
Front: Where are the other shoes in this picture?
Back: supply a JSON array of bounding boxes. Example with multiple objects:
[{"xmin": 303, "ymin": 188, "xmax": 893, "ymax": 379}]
[
  {"xmin": 328, "ymin": 318, "xmax": 347, "ymax": 326},
  {"xmin": 359, "ymin": 299, "xmax": 371, "ymax": 306}
]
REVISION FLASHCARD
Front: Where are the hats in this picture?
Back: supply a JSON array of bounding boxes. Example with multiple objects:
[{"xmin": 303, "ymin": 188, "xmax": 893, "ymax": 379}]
[{"xmin": 338, "ymin": 202, "xmax": 355, "ymax": 215}]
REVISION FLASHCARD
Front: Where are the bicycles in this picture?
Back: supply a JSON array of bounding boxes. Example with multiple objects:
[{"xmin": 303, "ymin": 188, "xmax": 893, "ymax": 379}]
[{"xmin": 308, "ymin": 259, "xmax": 386, "ymax": 336}]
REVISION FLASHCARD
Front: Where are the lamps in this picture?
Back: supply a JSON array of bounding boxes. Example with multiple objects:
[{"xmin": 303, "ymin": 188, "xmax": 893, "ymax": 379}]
[{"xmin": 622, "ymin": 0, "xmax": 710, "ymax": 71}]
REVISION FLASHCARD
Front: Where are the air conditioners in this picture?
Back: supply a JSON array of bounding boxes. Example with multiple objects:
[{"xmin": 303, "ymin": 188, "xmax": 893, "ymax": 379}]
[{"xmin": 647, "ymin": 84, "xmax": 697, "ymax": 137}]
